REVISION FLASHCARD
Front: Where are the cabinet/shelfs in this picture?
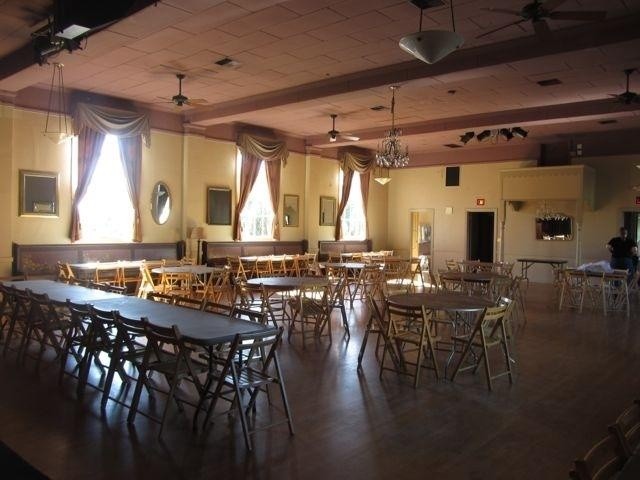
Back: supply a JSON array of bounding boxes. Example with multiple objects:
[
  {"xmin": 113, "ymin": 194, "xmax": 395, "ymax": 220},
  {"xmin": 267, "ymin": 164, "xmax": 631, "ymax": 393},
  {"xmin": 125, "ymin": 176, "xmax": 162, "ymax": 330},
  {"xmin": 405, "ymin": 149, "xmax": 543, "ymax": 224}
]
[{"xmin": 497, "ymin": 166, "xmax": 596, "ymax": 201}]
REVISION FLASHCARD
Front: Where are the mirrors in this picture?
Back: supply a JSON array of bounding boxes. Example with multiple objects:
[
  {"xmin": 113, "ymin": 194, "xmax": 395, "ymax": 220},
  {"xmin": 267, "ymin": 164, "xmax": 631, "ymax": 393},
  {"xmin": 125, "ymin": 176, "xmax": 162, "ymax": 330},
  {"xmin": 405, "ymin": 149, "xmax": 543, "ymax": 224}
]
[
  {"xmin": 19, "ymin": 169, "xmax": 61, "ymax": 218},
  {"xmin": 319, "ymin": 196, "xmax": 337, "ymax": 226},
  {"xmin": 206, "ymin": 187, "xmax": 232, "ymax": 226},
  {"xmin": 152, "ymin": 181, "xmax": 172, "ymax": 225},
  {"xmin": 284, "ymin": 194, "xmax": 300, "ymax": 226}
]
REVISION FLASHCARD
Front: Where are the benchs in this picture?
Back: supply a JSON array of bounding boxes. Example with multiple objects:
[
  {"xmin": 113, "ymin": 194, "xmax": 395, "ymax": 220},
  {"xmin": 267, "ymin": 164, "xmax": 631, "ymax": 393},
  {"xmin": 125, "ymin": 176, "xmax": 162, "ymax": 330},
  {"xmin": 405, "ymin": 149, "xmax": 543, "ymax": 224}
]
[
  {"xmin": 199, "ymin": 239, "xmax": 308, "ymax": 285},
  {"xmin": 13, "ymin": 241, "xmax": 188, "ymax": 294},
  {"xmin": 318, "ymin": 239, "xmax": 373, "ymax": 274}
]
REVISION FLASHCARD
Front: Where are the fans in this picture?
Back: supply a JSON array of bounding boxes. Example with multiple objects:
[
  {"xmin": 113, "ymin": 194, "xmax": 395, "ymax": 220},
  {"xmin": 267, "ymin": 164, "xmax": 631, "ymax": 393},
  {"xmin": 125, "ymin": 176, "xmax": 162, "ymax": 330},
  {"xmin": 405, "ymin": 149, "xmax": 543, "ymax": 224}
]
[
  {"xmin": 602, "ymin": 69, "xmax": 640, "ymax": 105},
  {"xmin": 145, "ymin": 73, "xmax": 216, "ymax": 114},
  {"xmin": 473, "ymin": 0, "xmax": 609, "ymax": 47},
  {"xmin": 308, "ymin": 113, "xmax": 360, "ymax": 143}
]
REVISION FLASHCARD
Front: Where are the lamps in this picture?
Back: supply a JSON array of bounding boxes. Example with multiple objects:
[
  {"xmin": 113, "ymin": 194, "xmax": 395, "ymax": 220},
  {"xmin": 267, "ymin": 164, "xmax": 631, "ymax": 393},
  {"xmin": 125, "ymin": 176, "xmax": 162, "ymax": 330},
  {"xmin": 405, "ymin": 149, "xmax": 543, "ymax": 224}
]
[
  {"xmin": 376, "ymin": 84, "xmax": 410, "ymax": 169},
  {"xmin": 372, "ymin": 141, "xmax": 393, "ymax": 186},
  {"xmin": 399, "ymin": 1, "xmax": 463, "ymax": 66},
  {"xmin": 40, "ymin": 60, "xmax": 74, "ymax": 144},
  {"xmin": 461, "ymin": 126, "xmax": 528, "ymax": 144}
]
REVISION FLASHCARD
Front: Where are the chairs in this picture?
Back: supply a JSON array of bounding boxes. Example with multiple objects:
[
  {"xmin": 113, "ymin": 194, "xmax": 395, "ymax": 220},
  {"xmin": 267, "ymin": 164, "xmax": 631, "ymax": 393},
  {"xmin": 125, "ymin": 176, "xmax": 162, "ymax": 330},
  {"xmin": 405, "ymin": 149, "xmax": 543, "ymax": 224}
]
[
  {"xmin": 516, "ymin": 258, "xmax": 640, "ymax": 318},
  {"xmin": 563, "ymin": 398, "xmax": 639, "ymax": 480},
  {"xmin": 2, "ymin": 250, "xmax": 528, "ymax": 452}
]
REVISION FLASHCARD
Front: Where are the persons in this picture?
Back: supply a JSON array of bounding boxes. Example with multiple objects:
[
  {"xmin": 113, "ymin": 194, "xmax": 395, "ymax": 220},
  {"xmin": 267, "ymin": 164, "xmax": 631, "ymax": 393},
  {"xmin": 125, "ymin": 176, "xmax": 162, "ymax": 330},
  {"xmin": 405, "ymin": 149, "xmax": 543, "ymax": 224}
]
[{"xmin": 606, "ymin": 227, "xmax": 638, "ymax": 304}]
